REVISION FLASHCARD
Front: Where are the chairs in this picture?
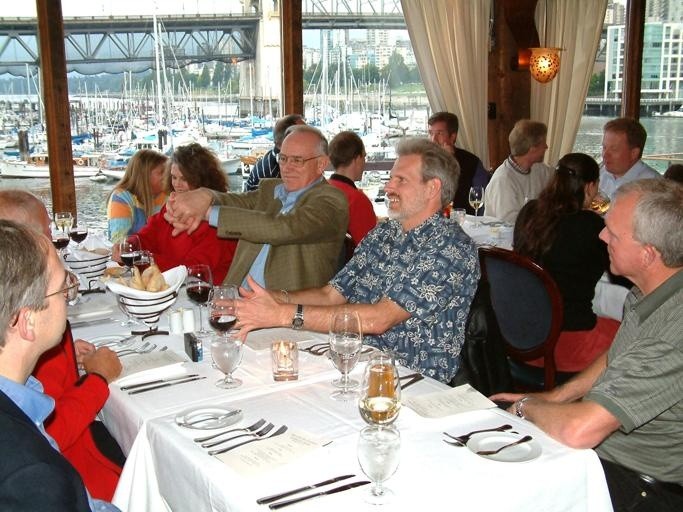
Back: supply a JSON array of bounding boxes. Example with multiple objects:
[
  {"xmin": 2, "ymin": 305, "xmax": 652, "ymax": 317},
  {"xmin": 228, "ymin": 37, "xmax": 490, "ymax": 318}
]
[
  {"xmin": 478, "ymin": 247, "xmax": 564, "ymax": 392},
  {"xmin": 342, "ymin": 229, "xmax": 355, "ymax": 265}
]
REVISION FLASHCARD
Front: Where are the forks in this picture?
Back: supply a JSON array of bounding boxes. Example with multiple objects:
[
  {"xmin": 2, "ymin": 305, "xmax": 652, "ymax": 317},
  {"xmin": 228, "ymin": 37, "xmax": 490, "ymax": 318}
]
[
  {"xmin": 443, "ymin": 424, "xmax": 512, "ymax": 443},
  {"xmin": 116, "ymin": 344, "xmax": 157, "ymax": 357},
  {"xmin": 201, "ymin": 423, "xmax": 275, "ymax": 448},
  {"xmin": 115, "ymin": 342, "xmax": 150, "ymax": 353},
  {"xmin": 442, "ymin": 431, "xmax": 519, "ymax": 447},
  {"xmin": 193, "ymin": 418, "xmax": 266, "ymax": 442},
  {"xmin": 207, "ymin": 424, "xmax": 288, "ymax": 456},
  {"xmin": 298, "ymin": 343, "xmax": 329, "ymax": 356}
]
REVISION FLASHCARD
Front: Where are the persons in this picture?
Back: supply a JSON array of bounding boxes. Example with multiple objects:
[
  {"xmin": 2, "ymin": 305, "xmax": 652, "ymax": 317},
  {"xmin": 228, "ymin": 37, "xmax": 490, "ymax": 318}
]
[
  {"xmin": 513, "ymin": 153, "xmax": 636, "ymax": 333},
  {"xmin": 107, "ymin": 151, "xmax": 168, "ymax": 243},
  {"xmin": 483, "ymin": 120, "xmax": 557, "ymax": 225},
  {"xmin": 213, "ymin": 140, "xmax": 482, "ymax": 385},
  {"xmin": 0, "ymin": 219, "xmax": 122, "ymax": 512},
  {"xmin": 426, "ymin": 112, "xmax": 486, "ymax": 215},
  {"xmin": 325, "ymin": 133, "xmax": 378, "ymax": 246},
  {"xmin": 598, "ymin": 118, "xmax": 666, "ymax": 199},
  {"xmin": 486, "ymin": 179, "xmax": 683, "ymax": 512},
  {"xmin": 164, "ymin": 125, "xmax": 348, "ymax": 290},
  {"xmin": 112, "ymin": 144, "xmax": 239, "ymax": 288},
  {"xmin": 0, "ymin": 192, "xmax": 129, "ymax": 503},
  {"xmin": 243, "ymin": 114, "xmax": 307, "ymax": 194}
]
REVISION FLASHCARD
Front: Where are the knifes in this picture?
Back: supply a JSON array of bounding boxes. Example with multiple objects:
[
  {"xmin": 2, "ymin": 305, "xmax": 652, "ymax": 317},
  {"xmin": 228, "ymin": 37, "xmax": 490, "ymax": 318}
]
[
  {"xmin": 255, "ymin": 474, "xmax": 356, "ymax": 505},
  {"xmin": 268, "ymin": 480, "xmax": 372, "ymax": 510},
  {"xmin": 127, "ymin": 376, "xmax": 207, "ymax": 395},
  {"xmin": 120, "ymin": 374, "xmax": 199, "ymax": 390}
]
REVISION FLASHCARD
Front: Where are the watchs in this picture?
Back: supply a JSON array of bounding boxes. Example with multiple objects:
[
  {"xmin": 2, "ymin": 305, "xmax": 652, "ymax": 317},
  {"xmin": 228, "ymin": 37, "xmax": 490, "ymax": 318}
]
[
  {"xmin": 514, "ymin": 396, "xmax": 533, "ymax": 421},
  {"xmin": 291, "ymin": 304, "xmax": 305, "ymax": 332}
]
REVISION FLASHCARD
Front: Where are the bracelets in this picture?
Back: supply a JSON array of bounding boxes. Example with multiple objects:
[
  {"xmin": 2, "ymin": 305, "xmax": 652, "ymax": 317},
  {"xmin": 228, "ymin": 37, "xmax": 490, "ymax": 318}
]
[{"xmin": 279, "ymin": 288, "xmax": 289, "ymax": 304}]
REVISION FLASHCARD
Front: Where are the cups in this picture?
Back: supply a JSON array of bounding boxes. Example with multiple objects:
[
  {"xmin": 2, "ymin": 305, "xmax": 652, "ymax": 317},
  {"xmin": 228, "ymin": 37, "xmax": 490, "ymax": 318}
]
[
  {"xmin": 209, "ymin": 330, "xmax": 243, "ymax": 389},
  {"xmin": 69, "ymin": 217, "xmax": 88, "ymax": 248},
  {"xmin": 54, "ymin": 212, "xmax": 72, "ymax": 231},
  {"xmin": 185, "ymin": 264, "xmax": 212, "ymax": 335},
  {"xmin": 119, "ymin": 235, "xmax": 141, "ymax": 277},
  {"xmin": 206, "ymin": 284, "xmax": 238, "ymax": 338},
  {"xmin": 329, "ymin": 313, "xmax": 361, "ymax": 388},
  {"xmin": 133, "ymin": 252, "xmax": 153, "ymax": 276},
  {"xmin": 452, "ymin": 208, "xmax": 466, "ymax": 227},
  {"xmin": 468, "ymin": 186, "xmax": 484, "ymax": 226},
  {"xmin": 360, "ymin": 176, "xmax": 379, "ymax": 207},
  {"xmin": 356, "ymin": 424, "xmax": 401, "ymax": 505},
  {"xmin": 49, "ymin": 229, "xmax": 73, "ymax": 258}
]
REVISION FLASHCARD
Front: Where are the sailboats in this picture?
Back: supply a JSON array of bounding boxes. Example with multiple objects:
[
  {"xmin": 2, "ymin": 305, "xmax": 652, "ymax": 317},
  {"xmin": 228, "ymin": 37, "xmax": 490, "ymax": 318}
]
[
  {"xmin": 1, "ymin": 80, "xmax": 40, "ymax": 160},
  {"xmin": 202, "ymin": 78, "xmax": 251, "ymax": 139},
  {"xmin": 101, "ymin": 26, "xmax": 242, "ymax": 181},
  {"xmin": 119, "ymin": 67, "xmax": 174, "ymax": 158},
  {"xmin": 0, "ymin": 63, "xmax": 99, "ymax": 176},
  {"xmin": 224, "ymin": 64, "xmax": 273, "ymax": 149}
]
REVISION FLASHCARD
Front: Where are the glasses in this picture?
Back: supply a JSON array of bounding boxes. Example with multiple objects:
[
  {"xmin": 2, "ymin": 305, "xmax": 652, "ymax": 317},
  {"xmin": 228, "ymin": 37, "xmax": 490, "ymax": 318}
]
[
  {"xmin": 275, "ymin": 151, "xmax": 326, "ymax": 169},
  {"xmin": 10, "ymin": 268, "xmax": 81, "ymax": 327}
]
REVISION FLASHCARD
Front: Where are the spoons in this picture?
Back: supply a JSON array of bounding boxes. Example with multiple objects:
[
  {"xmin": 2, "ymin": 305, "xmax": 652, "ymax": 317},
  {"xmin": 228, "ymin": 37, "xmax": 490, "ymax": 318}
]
[
  {"xmin": 176, "ymin": 408, "xmax": 241, "ymax": 428},
  {"xmin": 475, "ymin": 435, "xmax": 533, "ymax": 455}
]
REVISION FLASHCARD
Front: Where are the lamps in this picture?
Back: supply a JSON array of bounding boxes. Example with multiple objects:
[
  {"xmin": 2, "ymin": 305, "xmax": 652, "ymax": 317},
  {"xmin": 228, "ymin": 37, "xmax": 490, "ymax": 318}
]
[{"xmin": 528, "ymin": 46, "xmax": 562, "ymax": 84}]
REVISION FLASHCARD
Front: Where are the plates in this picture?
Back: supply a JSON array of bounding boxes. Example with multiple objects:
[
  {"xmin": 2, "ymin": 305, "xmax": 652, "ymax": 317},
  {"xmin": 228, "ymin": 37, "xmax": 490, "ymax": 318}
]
[
  {"xmin": 174, "ymin": 403, "xmax": 244, "ymax": 430},
  {"xmin": 84, "ymin": 334, "xmax": 135, "ymax": 351},
  {"xmin": 465, "ymin": 431, "xmax": 542, "ymax": 462}
]
